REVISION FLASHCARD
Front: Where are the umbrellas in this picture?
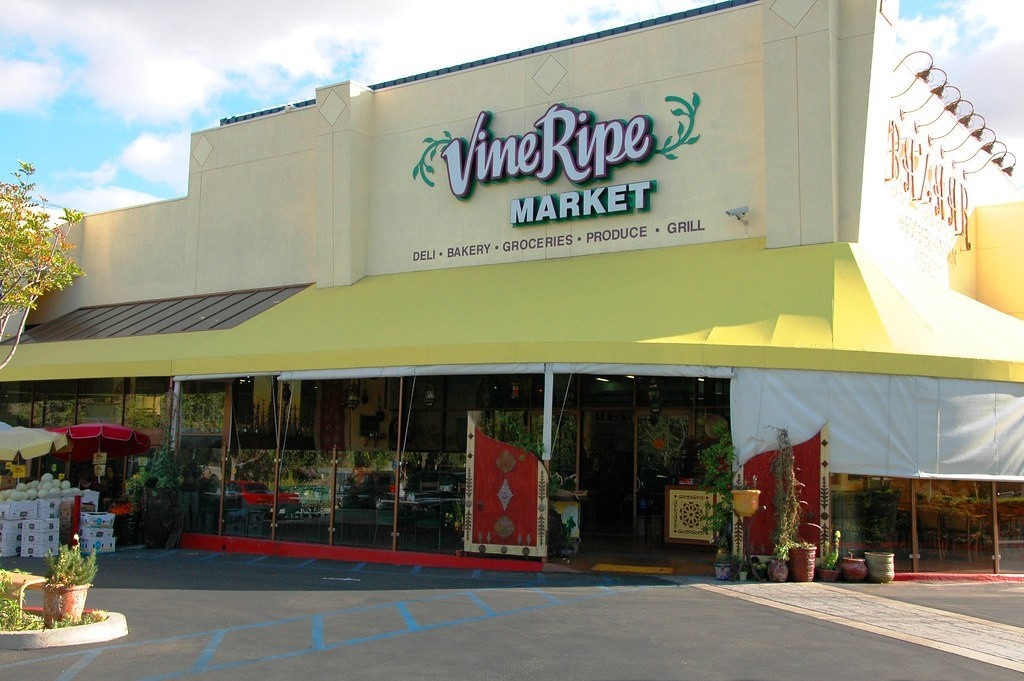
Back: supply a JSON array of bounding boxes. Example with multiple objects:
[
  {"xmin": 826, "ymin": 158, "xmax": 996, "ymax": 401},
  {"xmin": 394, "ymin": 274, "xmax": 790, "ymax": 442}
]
[
  {"xmin": 48, "ymin": 421, "xmax": 151, "ymax": 485},
  {"xmin": 0, "ymin": 426, "xmax": 67, "ymax": 484}
]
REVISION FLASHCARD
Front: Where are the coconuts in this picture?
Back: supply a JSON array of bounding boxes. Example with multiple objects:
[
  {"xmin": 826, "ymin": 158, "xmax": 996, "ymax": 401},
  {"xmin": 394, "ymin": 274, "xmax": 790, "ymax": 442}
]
[{"xmin": 0, "ymin": 473, "xmax": 81, "ymax": 501}]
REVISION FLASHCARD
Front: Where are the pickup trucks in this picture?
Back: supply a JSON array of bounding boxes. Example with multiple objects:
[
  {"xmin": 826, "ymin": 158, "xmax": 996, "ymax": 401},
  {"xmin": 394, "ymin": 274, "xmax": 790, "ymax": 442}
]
[{"xmin": 225, "ymin": 480, "xmax": 301, "ymax": 517}]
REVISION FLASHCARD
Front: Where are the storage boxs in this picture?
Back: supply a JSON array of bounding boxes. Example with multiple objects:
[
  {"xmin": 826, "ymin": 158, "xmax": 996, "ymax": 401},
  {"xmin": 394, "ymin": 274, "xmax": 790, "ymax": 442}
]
[{"xmin": 0, "ymin": 490, "xmax": 116, "ymax": 557}]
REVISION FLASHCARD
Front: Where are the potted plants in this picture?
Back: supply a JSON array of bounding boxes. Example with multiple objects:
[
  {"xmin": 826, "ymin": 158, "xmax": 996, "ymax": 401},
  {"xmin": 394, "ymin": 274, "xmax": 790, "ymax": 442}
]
[
  {"xmin": 856, "ymin": 480, "xmax": 901, "ymax": 582},
  {"xmin": 841, "ymin": 550, "xmax": 868, "ymax": 582},
  {"xmin": 817, "ymin": 530, "xmax": 841, "ymax": 581},
  {"xmin": 731, "ymin": 474, "xmax": 762, "ymax": 516},
  {"xmin": 768, "ymin": 545, "xmax": 791, "ymax": 582},
  {"xmin": 43, "ymin": 545, "xmax": 99, "ymax": 627},
  {"xmin": 712, "ymin": 536, "xmax": 731, "ymax": 580},
  {"xmin": 123, "ymin": 439, "xmax": 192, "ymax": 552},
  {"xmin": 738, "ymin": 559, "xmax": 749, "ymax": 581},
  {"xmin": 789, "ymin": 541, "xmax": 817, "ymax": 582}
]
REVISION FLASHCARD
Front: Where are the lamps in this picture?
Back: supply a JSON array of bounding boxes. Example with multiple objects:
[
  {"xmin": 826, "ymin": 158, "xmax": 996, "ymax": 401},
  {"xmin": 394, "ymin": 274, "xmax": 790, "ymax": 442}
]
[{"xmin": 890, "ymin": 49, "xmax": 1015, "ymax": 178}]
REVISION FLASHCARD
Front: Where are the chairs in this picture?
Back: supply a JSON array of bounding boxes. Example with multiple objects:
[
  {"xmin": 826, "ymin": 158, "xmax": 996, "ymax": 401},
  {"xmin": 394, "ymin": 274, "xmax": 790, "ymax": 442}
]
[
  {"xmin": 917, "ymin": 510, "xmax": 1024, "ymax": 563},
  {"xmin": 223, "ymin": 499, "xmax": 458, "ymax": 552}
]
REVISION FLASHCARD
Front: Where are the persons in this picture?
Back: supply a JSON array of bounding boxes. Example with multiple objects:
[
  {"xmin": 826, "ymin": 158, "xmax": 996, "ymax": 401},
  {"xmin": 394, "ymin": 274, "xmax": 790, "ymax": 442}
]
[
  {"xmin": 142, "ymin": 476, "xmax": 177, "ymax": 526},
  {"xmin": 179, "ymin": 464, "xmax": 219, "ymax": 534},
  {"xmin": 77, "ymin": 470, "xmax": 94, "ymax": 490}
]
